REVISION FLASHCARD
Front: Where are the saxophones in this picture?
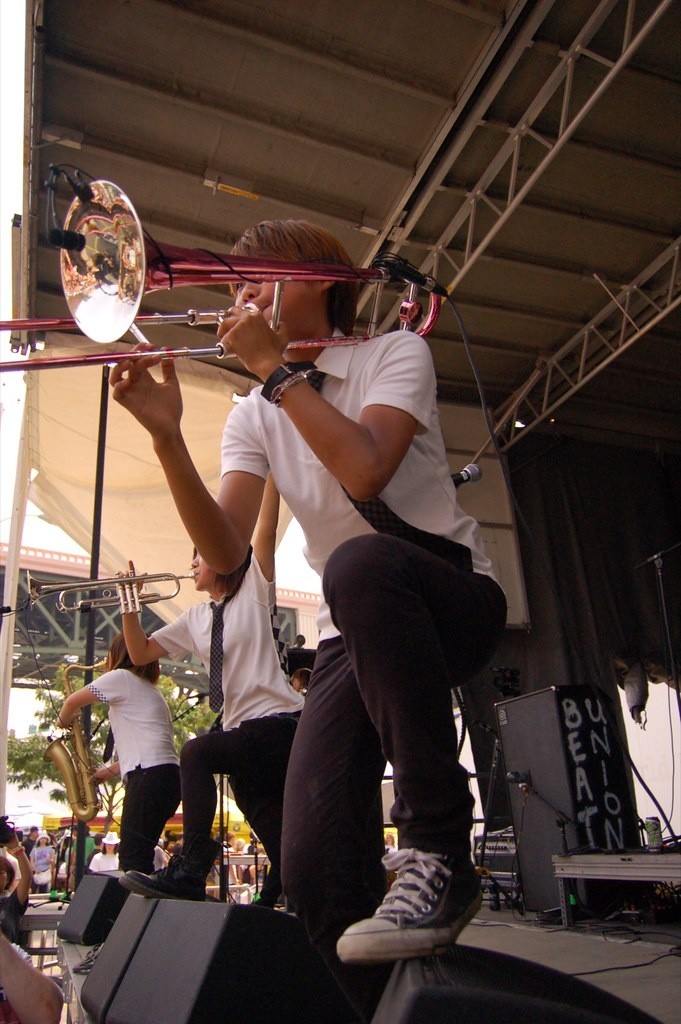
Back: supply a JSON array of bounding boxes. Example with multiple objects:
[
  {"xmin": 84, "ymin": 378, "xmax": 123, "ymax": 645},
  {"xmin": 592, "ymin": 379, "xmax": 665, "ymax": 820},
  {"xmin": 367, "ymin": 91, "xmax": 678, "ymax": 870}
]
[{"xmin": 42, "ymin": 658, "xmax": 107, "ymax": 822}]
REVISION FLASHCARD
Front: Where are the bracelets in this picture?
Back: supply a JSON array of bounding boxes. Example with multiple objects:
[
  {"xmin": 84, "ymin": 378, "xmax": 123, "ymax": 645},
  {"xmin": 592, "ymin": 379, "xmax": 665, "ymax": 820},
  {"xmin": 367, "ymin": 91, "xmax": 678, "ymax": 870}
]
[
  {"xmin": 261, "ymin": 360, "xmax": 318, "ymax": 402},
  {"xmin": 8, "ymin": 845, "xmax": 25, "ymax": 856},
  {"xmin": 270, "ymin": 370, "xmax": 311, "ymax": 408}
]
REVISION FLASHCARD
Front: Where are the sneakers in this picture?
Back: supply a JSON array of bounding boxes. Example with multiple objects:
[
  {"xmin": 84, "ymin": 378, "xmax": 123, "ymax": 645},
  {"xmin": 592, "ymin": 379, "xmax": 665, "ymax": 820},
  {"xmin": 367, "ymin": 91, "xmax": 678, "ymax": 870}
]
[{"xmin": 335, "ymin": 845, "xmax": 483, "ymax": 966}]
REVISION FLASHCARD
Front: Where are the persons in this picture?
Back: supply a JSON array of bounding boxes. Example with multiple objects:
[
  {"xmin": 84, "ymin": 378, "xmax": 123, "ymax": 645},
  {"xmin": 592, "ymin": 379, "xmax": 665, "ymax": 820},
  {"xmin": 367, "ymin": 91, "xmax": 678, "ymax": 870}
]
[
  {"xmin": 0, "ymin": 831, "xmax": 33, "ymax": 943},
  {"xmin": 111, "ymin": 218, "xmax": 509, "ymax": 963},
  {"xmin": 56, "ymin": 630, "xmax": 181, "ymax": 876},
  {"xmin": 117, "ymin": 472, "xmax": 306, "ymax": 906},
  {"xmin": 0, "ymin": 930, "xmax": 64, "ymax": 1024},
  {"xmin": 22, "ymin": 827, "xmax": 265, "ymax": 893},
  {"xmin": 385, "ymin": 835, "xmax": 397, "ymax": 854}
]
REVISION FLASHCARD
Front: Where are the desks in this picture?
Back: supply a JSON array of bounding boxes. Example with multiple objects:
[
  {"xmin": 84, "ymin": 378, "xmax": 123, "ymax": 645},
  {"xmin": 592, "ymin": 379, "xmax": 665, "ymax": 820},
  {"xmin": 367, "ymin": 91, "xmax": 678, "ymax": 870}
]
[{"xmin": 550, "ymin": 850, "xmax": 681, "ymax": 929}]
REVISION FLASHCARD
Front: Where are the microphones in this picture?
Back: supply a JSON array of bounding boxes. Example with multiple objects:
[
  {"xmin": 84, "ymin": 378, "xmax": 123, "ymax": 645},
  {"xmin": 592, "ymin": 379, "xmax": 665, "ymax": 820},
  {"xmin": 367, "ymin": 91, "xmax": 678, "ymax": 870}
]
[
  {"xmin": 48, "ymin": 736, "xmax": 53, "ymax": 743},
  {"xmin": 0, "ymin": 605, "xmax": 12, "ymax": 614},
  {"xmin": 395, "ymin": 264, "xmax": 447, "ymax": 298},
  {"xmin": 47, "ymin": 229, "xmax": 86, "ymax": 253},
  {"xmin": 450, "ymin": 463, "xmax": 483, "ymax": 488},
  {"xmin": 506, "ymin": 771, "xmax": 528, "ymax": 790}
]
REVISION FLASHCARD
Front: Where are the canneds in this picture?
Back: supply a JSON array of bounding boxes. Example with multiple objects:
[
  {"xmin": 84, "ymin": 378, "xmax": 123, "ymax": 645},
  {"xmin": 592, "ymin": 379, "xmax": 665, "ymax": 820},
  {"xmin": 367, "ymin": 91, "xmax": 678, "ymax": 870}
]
[
  {"xmin": 645, "ymin": 816, "xmax": 663, "ymax": 851},
  {"xmin": 489, "ymin": 883, "xmax": 501, "ymax": 910}
]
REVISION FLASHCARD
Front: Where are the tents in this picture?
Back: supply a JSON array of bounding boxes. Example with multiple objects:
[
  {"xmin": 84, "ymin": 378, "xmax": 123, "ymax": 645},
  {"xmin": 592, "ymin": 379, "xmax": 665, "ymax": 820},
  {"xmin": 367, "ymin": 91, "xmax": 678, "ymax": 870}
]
[{"xmin": 43, "ymin": 783, "xmax": 252, "ymax": 844}]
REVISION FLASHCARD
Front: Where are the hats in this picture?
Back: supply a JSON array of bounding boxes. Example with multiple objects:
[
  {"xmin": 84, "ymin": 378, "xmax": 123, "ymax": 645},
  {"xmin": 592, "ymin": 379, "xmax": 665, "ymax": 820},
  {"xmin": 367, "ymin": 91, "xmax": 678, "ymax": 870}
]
[
  {"xmin": 35, "ymin": 833, "xmax": 51, "ymax": 844},
  {"xmin": 101, "ymin": 831, "xmax": 121, "ymax": 844}
]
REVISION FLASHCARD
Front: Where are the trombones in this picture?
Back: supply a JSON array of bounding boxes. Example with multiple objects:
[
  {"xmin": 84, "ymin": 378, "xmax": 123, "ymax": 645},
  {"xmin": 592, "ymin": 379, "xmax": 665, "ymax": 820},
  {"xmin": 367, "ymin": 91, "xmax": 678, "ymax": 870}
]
[{"xmin": 0, "ymin": 174, "xmax": 441, "ymax": 373}]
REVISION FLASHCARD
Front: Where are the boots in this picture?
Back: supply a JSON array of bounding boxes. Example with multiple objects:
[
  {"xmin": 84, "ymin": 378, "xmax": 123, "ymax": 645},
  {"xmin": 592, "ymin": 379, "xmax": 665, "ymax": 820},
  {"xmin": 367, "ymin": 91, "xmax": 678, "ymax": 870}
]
[{"xmin": 119, "ymin": 836, "xmax": 221, "ymax": 901}]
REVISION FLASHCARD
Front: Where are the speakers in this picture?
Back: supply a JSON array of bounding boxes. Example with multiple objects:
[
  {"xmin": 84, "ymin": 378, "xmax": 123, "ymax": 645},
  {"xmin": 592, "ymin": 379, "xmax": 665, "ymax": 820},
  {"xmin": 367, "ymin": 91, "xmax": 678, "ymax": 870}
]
[
  {"xmin": 57, "ymin": 874, "xmax": 665, "ymax": 1024},
  {"xmin": 495, "ymin": 683, "xmax": 641, "ymax": 914}
]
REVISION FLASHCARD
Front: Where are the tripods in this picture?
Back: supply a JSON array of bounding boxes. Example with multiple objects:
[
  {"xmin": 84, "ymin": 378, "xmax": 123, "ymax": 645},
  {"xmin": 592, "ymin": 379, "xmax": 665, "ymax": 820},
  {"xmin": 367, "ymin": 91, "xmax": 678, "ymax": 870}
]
[{"xmin": 27, "ymin": 843, "xmax": 71, "ymax": 911}]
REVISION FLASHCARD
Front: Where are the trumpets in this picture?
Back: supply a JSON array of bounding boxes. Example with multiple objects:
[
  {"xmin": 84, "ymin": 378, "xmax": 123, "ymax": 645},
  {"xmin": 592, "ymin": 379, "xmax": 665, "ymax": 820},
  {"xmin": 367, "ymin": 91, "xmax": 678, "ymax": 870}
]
[{"xmin": 23, "ymin": 569, "xmax": 195, "ymax": 616}]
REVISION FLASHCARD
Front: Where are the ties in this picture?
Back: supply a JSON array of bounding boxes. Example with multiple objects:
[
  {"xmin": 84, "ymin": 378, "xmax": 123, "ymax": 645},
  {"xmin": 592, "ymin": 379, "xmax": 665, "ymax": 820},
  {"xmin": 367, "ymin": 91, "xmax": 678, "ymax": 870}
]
[
  {"xmin": 306, "ymin": 369, "xmax": 474, "ymax": 568},
  {"xmin": 209, "ymin": 596, "xmax": 234, "ymax": 713},
  {"xmin": 103, "ymin": 727, "xmax": 114, "ymax": 763}
]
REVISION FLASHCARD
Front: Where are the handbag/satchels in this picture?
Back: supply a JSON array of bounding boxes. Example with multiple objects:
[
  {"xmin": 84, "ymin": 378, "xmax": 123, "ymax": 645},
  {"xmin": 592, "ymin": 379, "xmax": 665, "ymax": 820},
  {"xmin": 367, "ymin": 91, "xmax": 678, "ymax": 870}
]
[{"xmin": 33, "ymin": 868, "xmax": 51, "ymax": 885}]
